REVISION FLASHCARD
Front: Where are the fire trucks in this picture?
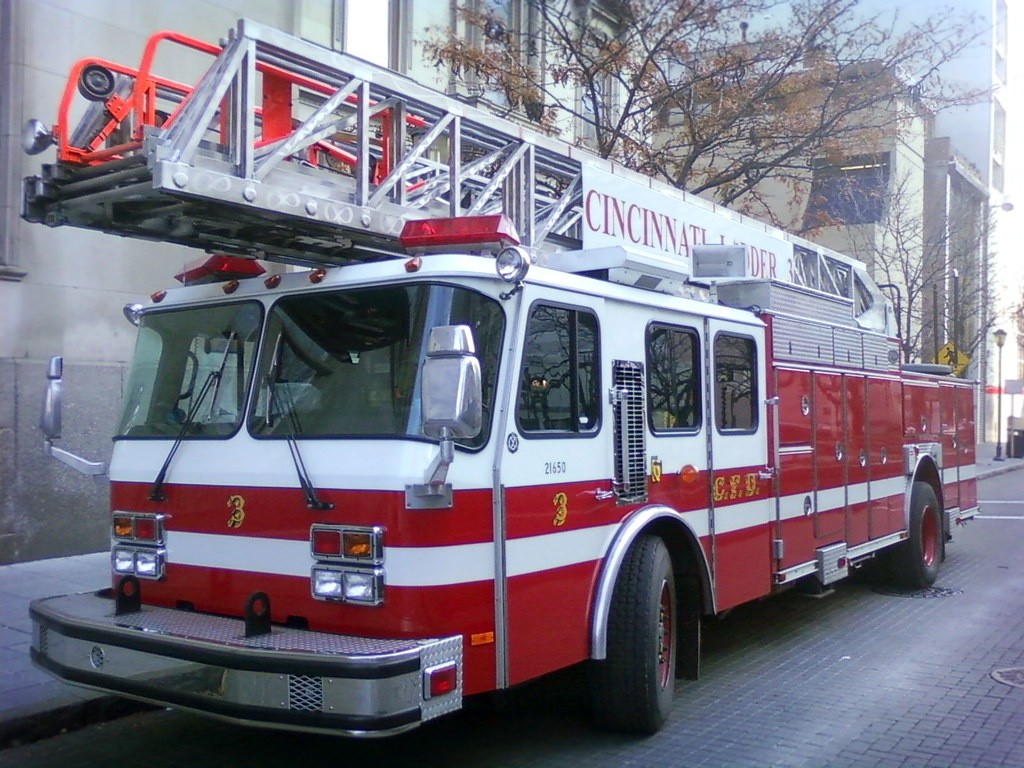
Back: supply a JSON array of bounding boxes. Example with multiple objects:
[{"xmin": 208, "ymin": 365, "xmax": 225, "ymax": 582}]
[{"xmin": 19, "ymin": 15, "xmax": 980, "ymax": 739}]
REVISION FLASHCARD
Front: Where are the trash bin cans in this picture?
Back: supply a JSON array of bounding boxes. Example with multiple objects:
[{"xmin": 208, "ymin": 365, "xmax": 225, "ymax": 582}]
[{"xmin": 1006, "ymin": 428, "xmax": 1024, "ymax": 458}]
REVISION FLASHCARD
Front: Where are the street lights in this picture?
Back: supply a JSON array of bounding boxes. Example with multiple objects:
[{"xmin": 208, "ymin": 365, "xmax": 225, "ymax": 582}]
[
  {"xmin": 983, "ymin": 200, "xmax": 1014, "ymax": 444},
  {"xmin": 993, "ymin": 328, "xmax": 1007, "ymax": 460}
]
